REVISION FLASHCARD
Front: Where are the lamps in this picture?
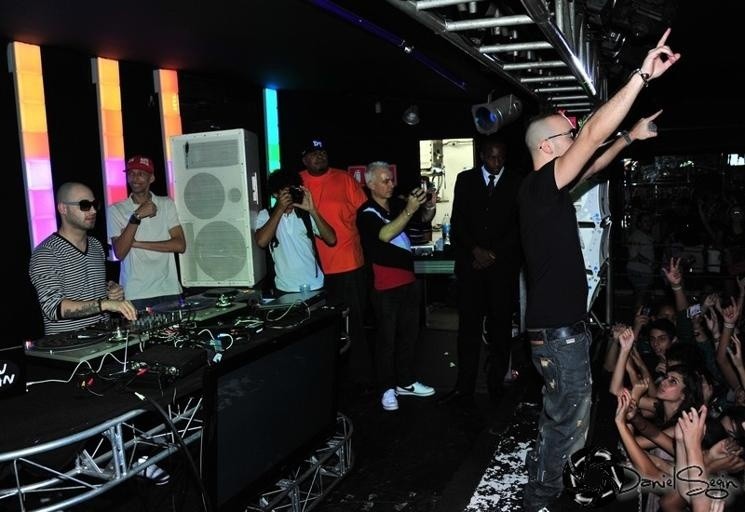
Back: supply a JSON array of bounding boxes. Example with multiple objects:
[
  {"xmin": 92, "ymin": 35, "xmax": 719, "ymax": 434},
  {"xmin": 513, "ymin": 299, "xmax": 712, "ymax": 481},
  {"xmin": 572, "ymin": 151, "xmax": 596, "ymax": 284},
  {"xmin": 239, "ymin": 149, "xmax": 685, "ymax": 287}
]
[{"xmin": 470, "ymin": 91, "xmax": 524, "ymax": 136}]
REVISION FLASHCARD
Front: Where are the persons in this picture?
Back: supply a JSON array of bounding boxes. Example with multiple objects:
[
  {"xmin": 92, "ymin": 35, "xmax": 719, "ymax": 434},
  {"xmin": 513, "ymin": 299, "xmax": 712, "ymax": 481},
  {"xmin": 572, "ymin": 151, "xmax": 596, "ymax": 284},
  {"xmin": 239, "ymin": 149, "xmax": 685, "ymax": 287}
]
[
  {"xmin": 354, "ymin": 160, "xmax": 439, "ymax": 413},
  {"xmin": 106, "ymin": 153, "xmax": 186, "ymax": 311},
  {"xmin": 297, "ymin": 136, "xmax": 370, "ymax": 330},
  {"xmin": 519, "ymin": 27, "xmax": 682, "ymax": 511},
  {"xmin": 29, "ymin": 180, "xmax": 172, "ymax": 511},
  {"xmin": 436, "ymin": 137, "xmax": 524, "ymax": 407},
  {"xmin": 254, "ymin": 168, "xmax": 337, "ymax": 297},
  {"xmin": 598, "ymin": 165, "xmax": 745, "ymax": 510}
]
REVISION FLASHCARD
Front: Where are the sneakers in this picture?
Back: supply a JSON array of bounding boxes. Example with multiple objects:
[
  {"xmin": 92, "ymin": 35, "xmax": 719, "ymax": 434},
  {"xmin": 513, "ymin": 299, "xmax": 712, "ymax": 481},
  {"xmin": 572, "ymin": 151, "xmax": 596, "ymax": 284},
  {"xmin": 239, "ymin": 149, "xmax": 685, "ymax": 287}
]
[
  {"xmin": 396, "ymin": 381, "xmax": 436, "ymax": 398},
  {"xmin": 382, "ymin": 388, "xmax": 399, "ymax": 411}
]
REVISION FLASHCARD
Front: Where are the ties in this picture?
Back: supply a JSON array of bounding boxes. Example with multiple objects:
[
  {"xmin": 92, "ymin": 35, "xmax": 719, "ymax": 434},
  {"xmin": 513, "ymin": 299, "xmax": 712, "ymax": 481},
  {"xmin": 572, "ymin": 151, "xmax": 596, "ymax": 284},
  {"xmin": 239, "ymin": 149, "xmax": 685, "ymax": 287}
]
[{"xmin": 488, "ymin": 175, "xmax": 496, "ymax": 198}]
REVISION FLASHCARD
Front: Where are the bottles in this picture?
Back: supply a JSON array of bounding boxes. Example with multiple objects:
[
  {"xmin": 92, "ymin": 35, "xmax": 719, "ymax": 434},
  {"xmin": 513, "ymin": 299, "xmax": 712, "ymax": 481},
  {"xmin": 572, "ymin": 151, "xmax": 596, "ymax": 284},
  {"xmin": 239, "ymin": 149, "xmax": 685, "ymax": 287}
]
[{"xmin": 442, "ymin": 212, "xmax": 452, "ymax": 244}]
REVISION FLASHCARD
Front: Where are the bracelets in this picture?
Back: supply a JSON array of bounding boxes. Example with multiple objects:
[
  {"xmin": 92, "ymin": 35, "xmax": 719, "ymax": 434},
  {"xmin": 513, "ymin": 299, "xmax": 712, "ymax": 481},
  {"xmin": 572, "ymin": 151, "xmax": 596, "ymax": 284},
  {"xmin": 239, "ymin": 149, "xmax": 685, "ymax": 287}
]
[
  {"xmin": 623, "ymin": 131, "xmax": 636, "ymax": 146},
  {"xmin": 133, "ymin": 212, "xmax": 141, "ymax": 220},
  {"xmin": 130, "ymin": 215, "xmax": 140, "ymax": 226},
  {"xmin": 404, "ymin": 208, "xmax": 414, "ymax": 217},
  {"xmin": 636, "ymin": 67, "xmax": 652, "ymax": 88},
  {"xmin": 98, "ymin": 298, "xmax": 105, "ymax": 314},
  {"xmin": 424, "ymin": 203, "xmax": 436, "ymax": 210}
]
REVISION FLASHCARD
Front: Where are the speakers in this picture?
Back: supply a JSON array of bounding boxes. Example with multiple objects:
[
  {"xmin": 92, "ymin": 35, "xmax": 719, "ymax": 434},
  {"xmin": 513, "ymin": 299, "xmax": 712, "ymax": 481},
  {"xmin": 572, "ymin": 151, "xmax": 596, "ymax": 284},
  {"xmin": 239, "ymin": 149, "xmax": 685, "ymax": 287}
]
[{"xmin": 170, "ymin": 125, "xmax": 270, "ymax": 287}]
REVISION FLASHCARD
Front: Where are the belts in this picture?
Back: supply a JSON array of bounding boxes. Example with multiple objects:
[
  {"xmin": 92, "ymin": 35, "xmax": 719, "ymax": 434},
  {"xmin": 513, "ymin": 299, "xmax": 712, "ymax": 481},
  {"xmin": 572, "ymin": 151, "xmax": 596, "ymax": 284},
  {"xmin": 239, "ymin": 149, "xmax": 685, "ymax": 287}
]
[{"xmin": 527, "ymin": 321, "xmax": 588, "ymax": 343}]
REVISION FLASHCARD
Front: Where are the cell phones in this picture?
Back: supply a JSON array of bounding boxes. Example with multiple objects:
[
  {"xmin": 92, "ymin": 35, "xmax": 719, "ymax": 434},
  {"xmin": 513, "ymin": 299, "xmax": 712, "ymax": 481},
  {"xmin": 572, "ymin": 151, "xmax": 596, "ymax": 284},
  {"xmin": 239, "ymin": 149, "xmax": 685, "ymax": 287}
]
[{"xmin": 641, "ymin": 304, "xmax": 652, "ymax": 316}]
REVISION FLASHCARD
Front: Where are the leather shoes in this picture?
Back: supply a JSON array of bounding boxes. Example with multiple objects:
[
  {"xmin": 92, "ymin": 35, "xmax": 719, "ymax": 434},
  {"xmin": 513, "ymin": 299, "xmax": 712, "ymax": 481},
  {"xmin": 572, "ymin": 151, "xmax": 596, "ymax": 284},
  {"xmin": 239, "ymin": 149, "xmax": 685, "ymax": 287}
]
[
  {"xmin": 489, "ymin": 380, "xmax": 504, "ymax": 401},
  {"xmin": 435, "ymin": 386, "xmax": 475, "ymax": 408}
]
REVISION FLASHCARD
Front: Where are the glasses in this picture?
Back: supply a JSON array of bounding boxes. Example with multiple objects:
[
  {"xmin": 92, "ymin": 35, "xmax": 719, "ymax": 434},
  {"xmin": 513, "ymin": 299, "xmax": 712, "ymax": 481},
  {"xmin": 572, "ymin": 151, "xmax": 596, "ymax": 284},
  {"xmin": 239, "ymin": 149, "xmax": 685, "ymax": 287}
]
[
  {"xmin": 539, "ymin": 127, "xmax": 579, "ymax": 149},
  {"xmin": 62, "ymin": 199, "xmax": 101, "ymax": 211}
]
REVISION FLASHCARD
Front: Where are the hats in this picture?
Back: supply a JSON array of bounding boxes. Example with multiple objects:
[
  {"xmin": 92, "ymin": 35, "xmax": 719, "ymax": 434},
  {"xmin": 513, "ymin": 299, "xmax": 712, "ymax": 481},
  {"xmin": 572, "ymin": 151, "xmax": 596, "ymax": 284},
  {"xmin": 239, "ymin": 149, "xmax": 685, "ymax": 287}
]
[
  {"xmin": 122, "ymin": 155, "xmax": 155, "ymax": 173},
  {"xmin": 304, "ymin": 136, "xmax": 327, "ymax": 153}
]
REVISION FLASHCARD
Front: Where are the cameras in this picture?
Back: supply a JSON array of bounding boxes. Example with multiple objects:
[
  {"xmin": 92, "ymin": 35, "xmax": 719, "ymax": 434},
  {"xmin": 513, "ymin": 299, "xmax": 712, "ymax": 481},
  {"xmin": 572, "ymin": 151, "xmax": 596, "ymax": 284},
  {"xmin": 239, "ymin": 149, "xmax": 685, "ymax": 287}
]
[
  {"xmin": 694, "ymin": 395, "xmax": 729, "ymax": 422},
  {"xmin": 288, "ymin": 185, "xmax": 304, "ymax": 204},
  {"xmin": 407, "ymin": 188, "xmax": 433, "ymax": 202}
]
